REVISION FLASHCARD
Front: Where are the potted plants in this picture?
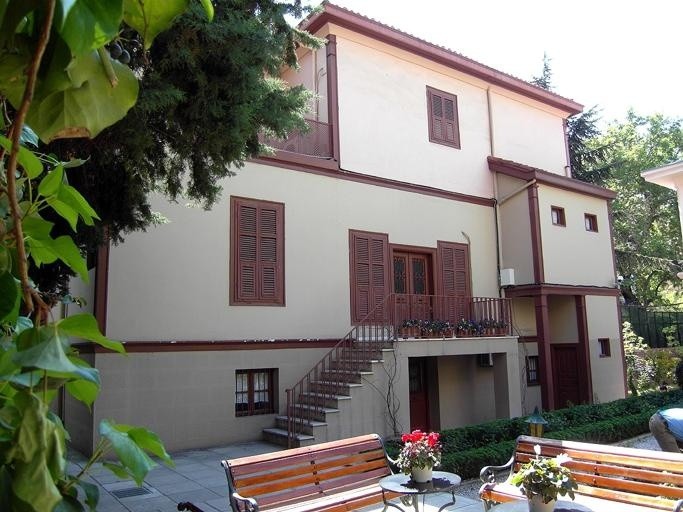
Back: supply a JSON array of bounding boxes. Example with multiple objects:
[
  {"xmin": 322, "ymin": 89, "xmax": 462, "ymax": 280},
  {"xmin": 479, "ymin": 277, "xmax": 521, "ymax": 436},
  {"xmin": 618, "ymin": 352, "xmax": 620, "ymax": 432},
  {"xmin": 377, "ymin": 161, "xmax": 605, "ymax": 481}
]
[
  {"xmin": 397, "ymin": 317, "xmax": 508, "ymax": 338},
  {"xmin": 509, "ymin": 444, "xmax": 578, "ymax": 512}
]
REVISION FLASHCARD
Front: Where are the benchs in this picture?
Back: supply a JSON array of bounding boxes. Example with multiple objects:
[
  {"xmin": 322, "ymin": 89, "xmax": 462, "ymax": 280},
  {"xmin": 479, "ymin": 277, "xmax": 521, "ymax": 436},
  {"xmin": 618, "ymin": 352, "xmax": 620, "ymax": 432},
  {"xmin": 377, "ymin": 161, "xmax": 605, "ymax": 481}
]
[
  {"xmin": 220, "ymin": 433, "xmax": 408, "ymax": 512},
  {"xmin": 479, "ymin": 434, "xmax": 683, "ymax": 512}
]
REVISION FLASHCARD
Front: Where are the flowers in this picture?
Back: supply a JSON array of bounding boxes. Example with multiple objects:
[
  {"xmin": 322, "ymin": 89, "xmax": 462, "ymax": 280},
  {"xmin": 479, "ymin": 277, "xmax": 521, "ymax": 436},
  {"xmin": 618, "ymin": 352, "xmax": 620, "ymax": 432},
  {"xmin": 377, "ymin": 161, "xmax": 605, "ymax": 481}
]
[{"xmin": 397, "ymin": 428, "xmax": 443, "ymax": 475}]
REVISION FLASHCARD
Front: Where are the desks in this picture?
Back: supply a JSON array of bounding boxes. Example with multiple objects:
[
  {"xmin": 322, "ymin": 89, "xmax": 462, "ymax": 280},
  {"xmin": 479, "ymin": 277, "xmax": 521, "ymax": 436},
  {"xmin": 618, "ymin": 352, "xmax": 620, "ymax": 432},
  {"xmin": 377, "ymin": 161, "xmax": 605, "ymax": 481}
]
[
  {"xmin": 378, "ymin": 471, "xmax": 463, "ymax": 512},
  {"xmin": 486, "ymin": 500, "xmax": 593, "ymax": 512}
]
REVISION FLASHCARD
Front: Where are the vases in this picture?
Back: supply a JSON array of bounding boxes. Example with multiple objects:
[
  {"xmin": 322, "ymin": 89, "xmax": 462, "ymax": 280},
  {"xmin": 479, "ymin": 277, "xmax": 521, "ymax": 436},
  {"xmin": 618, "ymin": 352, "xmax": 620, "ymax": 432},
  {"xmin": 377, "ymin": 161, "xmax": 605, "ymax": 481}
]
[{"xmin": 411, "ymin": 465, "xmax": 432, "ymax": 482}]
